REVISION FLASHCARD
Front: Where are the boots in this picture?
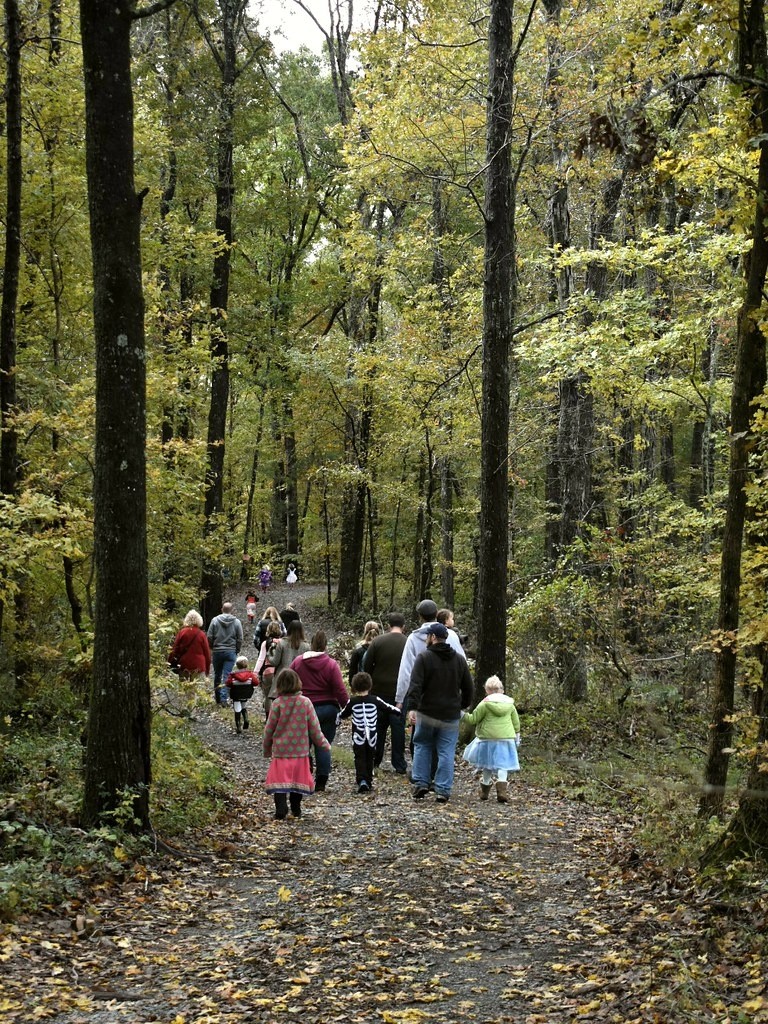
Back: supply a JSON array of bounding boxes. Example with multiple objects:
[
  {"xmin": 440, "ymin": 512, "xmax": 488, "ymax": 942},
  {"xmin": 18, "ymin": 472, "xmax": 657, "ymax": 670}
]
[
  {"xmin": 243, "ymin": 713, "xmax": 249, "ymax": 729},
  {"xmin": 479, "ymin": 783, "xmax": 493, "ymax": 800},
  {"xmin": 315, "ymin": 775, "xmax": 328, "ymax": 792},
  {"xmin": 289, "ymin": 793, "xmax": 303, "ymax": 816},
  {"xmin": 274, "ymin": 793, "xmax": 288, "ymax": 819},
  {"xmin": 496, "ymin": 782, "xmax": 508, "ymax": 803},
  {"xmin": 236, "ymin": 720, "xmax": 242, "ymax": 733}
]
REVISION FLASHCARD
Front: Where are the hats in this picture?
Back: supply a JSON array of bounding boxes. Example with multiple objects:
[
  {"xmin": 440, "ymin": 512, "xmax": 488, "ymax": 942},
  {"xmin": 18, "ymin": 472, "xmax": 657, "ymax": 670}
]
[
  {"xmin": 416, "ymin": 598, "xmax": 438, "ymax": 615},
  {"xmin": 421, "ymin": 624, "xmax": 448, "ymax": 639}
]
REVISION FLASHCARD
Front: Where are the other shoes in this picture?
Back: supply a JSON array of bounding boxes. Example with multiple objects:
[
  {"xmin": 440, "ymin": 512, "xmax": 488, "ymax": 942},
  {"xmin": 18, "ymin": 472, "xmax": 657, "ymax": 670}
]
[
  {"xmin": 436, "ymin": 794, "xmax": 447, "ymax": 802},
  {"xmin": 413, "ymin": 787, "xmax": 428, "ymax": 798},
  {"xmin": 396, "ymin": 768, "xmax": 406, "ymax": 774},
  {"xmin": 358, "ymin": 780, "xmax": 369, "ymax": 793},
  {"xmin": 221, "ymin": 701, "xmax": 230, "ymax": 708}
]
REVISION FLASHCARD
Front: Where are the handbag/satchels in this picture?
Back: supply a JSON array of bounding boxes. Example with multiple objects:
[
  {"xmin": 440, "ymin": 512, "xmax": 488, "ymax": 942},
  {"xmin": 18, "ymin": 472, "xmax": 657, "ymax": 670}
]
[{"xmin": 171, "ymin": 660, "xmax": 182, "ymax": 674}]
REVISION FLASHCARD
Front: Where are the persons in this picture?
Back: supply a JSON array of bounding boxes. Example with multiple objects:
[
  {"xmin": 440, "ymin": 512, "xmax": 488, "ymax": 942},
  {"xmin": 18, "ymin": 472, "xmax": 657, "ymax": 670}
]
[
  {"xmin": 253, "ymin": 621, "xmax": 285, "ymax": 721},
  {"xmin": 278, "ymin": 602, "xmax": 300, "ymax": 630},
  {"xmin": 253, "ymin": 606, "xmax": 287, "ymax": 653},
  {"xmin": 393, "ymin": 599, "xmax": 466, "ymax": 784},
  {"xmin": 437, "ymin": 609, "xmax": 455, "ymax": 628},
  {"xmin": 460, "ymin": 674, "xmax": 520, "ymax": 803},
  {"xmin": 257, "ymin": 570, "xmax": 272, "ymax": 594},
  {"xmin": 263, "ymin": 620, "xmax": 310, "ymax": 699},
  {"xmin": 262, "ymin": 668, "xmax": 330, "ymax": 820},
  {"xmin": 349, "ymin": 621, "xmax": 379, "ymax": 687},
  {"xmin": 168, "ymin": 609, "xmax": 211, "ymax": 719},
  {"xmin": 287, "ymin": 563, "xmax": 297, "ymax": 590},
  {"xmin": 335, "ymin": 672, "xmax": 403, "ymax": 794},
  {"xmin": 225, "ymin": 656, "xmax": 260, "ymax": 732},
  {"xmin": 406, "ymin": 624, "xmax": 474, "ymax": 802},
  {"xmin": 289, "ymin": 630, "xmax": 351, "ymax": 792},
  {"xmin": 245, "ymin": 589, "xmax": 260, "ymax": 624},
  {"xmin": 362, "ymin": 613, "xmax": 408, "ymax": 774},
  {"xmin": 205, "ymin": 603, "xmax": 243, "ymax": 707}
]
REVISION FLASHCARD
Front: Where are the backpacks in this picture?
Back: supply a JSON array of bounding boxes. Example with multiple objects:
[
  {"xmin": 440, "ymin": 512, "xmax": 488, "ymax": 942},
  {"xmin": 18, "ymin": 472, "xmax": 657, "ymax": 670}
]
[{"xmin": 260, "ymin": 638, "xmax": 276, "ymax": 684}]
